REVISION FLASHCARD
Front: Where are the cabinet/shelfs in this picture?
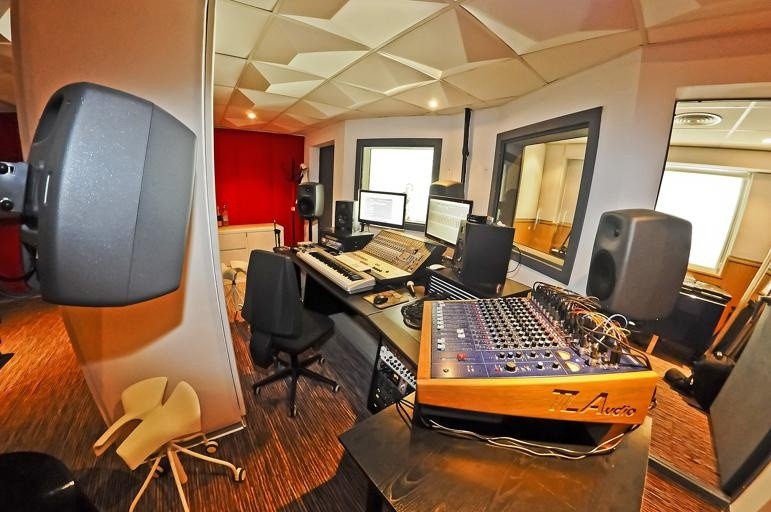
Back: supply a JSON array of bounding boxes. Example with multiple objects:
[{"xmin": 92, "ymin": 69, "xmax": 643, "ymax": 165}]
[{"xmin": 218, "ymin": 223, "xmax": 284, "ymax": 285}]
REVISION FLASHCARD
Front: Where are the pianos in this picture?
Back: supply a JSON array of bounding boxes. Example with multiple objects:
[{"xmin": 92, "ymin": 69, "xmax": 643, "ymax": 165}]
[{"xmin": 296, "ymin": 247, "xmax": 376, "ymax": 294}]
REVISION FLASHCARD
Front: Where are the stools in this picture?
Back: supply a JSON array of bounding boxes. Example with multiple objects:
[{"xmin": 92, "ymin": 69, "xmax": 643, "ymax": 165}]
[
  {"xmin": 93, "ymin": 377, "xmax": 246, "ymax": 512},
  {"xmin": 222, "ymin": 260, "xmax": 247, "ymax": 324}
]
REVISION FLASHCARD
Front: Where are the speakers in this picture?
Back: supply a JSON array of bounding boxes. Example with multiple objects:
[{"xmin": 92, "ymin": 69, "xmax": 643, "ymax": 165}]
[
  {"xmin": 18, "ymin": 80, "xmax": 196, "ymax": 309},
  {"xmin": 298, "ymin": 182, "xmax": 324, "ymax": 218},
  {"xmin": 429, "ymin": 180, "xmax": 464, "ymax": 198},
  {"xmin": 585, "ymin": 208, "xmax": 691, "ymax": 323},
  {"xmin": 335, "ymin": 200, "xmax": 358, "ymax": 234},
  {"xmin": 453, "ymin": 220, "xmax": 516, "ymax": 292}
]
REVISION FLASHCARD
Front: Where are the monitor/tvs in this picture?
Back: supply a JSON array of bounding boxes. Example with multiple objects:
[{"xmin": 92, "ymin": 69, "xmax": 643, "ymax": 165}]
[
  {"xmin": 357, "ymin": 189, "xmax": 406, "ymax": 230},
  {"xmin": 424, "ymin": 195, "xmax": 473, "ymax": 247}
]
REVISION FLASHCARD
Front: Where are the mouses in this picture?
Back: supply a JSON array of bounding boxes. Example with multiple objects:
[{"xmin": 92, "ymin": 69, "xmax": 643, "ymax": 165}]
[{"xmin": 374, "ymin": 294, "xmax": 388, "ymax": 304}]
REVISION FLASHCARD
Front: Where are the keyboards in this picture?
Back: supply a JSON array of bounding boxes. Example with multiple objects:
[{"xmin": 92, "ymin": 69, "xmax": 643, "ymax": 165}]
[{"xmin": 335, "ymin": 253, "xmax": 372, "ymax": 272}]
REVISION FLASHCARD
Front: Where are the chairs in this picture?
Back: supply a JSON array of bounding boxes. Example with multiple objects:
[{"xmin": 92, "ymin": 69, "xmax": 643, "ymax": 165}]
[{"xmin": 241, "ymin": 250, "xmax": 341, "ymax": 417}]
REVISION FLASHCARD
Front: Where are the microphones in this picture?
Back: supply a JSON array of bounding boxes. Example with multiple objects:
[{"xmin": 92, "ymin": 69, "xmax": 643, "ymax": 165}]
[{"xmin": 406, "ymin": 280, "xmax": 416, "ymax": 296}]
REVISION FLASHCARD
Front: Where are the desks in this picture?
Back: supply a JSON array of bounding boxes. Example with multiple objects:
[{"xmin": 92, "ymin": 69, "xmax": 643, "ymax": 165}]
[{"xmin": 273, "ymin": 247, "xmax": 653, "ymax": 511}]
